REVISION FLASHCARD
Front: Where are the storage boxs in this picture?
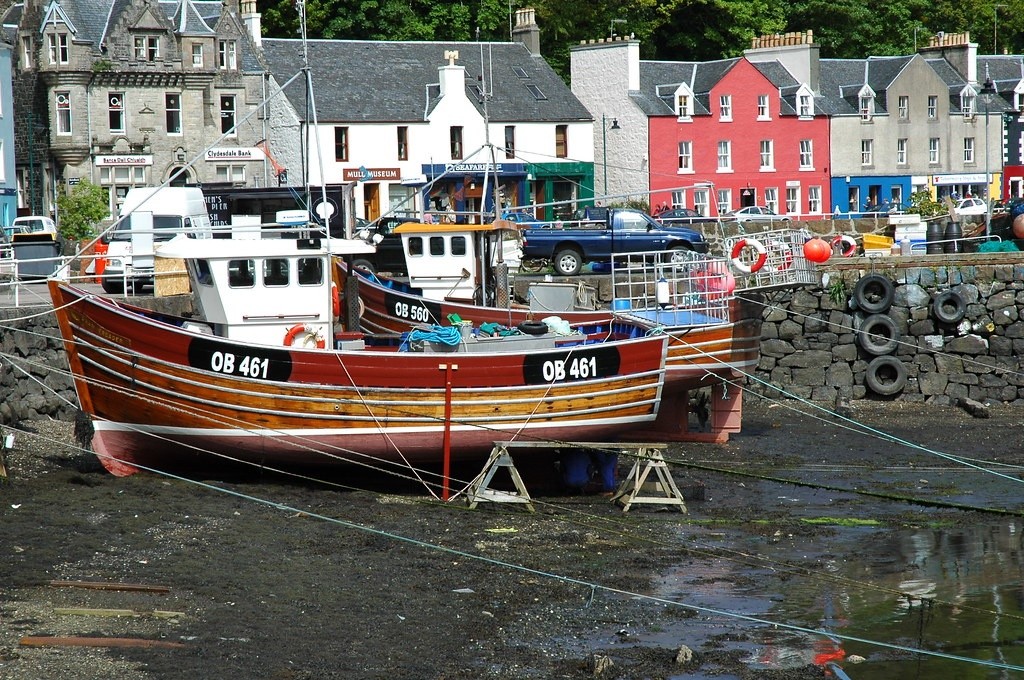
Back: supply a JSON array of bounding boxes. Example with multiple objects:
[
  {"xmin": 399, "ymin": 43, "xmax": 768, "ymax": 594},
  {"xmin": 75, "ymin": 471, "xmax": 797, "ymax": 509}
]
[
  {"xmin": 896, "ymin": 239, "xmax": 926, "ymax": 250},
  {"xmin": 895, "ymin": 231, "xmax": 926, "ymax": 239},
  {"xmin": 895, "ymin": 222, "xmax": 928, "ymax": 231},
  {"xmin": 888, "ymin": 214, "xmax": 920, "ymax": 225}
]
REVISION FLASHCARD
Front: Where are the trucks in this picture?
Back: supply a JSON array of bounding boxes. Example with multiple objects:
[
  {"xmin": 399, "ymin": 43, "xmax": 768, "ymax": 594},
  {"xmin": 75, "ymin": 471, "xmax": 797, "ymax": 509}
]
[
  {"xmin": 201, "ymin": 187, "xmax": 355, "ymax": 281},
  {"xmin": 102, "ymin": 186, "xmax": 213, "ymax": 295}
]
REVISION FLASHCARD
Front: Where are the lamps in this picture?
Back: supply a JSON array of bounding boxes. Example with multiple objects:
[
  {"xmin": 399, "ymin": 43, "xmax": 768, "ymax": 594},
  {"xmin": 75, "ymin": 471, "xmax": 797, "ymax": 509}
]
[
  {"xmin": 846, "ymin": 175, "xmax": 850, "ymax": 183},
  {"xmin": 747, "ymin": 182, "xmax": 751, "ymax": 187}
]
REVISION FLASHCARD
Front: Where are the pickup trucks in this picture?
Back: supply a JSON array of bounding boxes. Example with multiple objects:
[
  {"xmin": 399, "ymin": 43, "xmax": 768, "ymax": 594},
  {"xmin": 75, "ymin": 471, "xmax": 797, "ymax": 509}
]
[
  {"xmin": 351, "ymin": 218, "xmax": 465, "ymax": 275},
  {"xmin": 520, "ymin": 208, "xmax": 710, "ymax": 276}
]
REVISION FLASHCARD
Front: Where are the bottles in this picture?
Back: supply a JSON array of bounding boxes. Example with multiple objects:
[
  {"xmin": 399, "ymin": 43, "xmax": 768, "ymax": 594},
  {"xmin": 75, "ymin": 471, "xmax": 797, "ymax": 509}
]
[
  {"xmin": 900, "ymin": 236, "xmax": 910, "ymax": 256},
  {"xmin": 891, "ymin": 243, "xmax": 900, "ymax": 256}
]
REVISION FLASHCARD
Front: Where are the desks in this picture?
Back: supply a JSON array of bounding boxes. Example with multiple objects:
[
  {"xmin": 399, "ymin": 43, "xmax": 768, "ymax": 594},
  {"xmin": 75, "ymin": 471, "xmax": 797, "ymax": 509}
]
[{"xmin": 553, "ymin": 329, "xmax": 587, "ymax": 348}]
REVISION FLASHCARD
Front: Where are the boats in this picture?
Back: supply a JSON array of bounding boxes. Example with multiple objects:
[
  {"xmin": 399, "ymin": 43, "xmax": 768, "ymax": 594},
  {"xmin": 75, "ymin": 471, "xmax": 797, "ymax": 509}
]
[
  {"xmin": 47, "ymin": 0, "xmax": 668, "ymax": 479},
  {"xmin": 393, "ymin": 43, "xmax": 763, "ymax": 442}
]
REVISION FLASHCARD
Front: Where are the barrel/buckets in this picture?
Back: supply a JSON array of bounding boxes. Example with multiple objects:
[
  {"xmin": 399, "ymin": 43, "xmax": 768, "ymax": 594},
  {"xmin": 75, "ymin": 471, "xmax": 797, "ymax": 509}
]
[
  {"xmin": 944, "ymin": 221, "xmax": 963, "ymax": 253},
  {"xmin": 610, "ymin": 299, "xmax": 630, "ymax": 311},
  {"xmin": 458, "ymin": 324, "xmax": 472, "ymax": 338},
  {"xmin": 927, "ymin": 222, "xmax": 944, "ymax": 254},
  {"xmin": 397, "ymin": 332, "xmax": 410, "ymax": 352}
]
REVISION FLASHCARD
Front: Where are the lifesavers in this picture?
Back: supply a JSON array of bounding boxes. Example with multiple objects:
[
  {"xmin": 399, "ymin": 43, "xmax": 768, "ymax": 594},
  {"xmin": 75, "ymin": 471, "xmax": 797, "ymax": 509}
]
[
  {"xmin": 730, "ymin": 238, "xmax": 766, "ymax": 273},
  {"xmin": 332, "ymin": 281, "xmax": 341, "ymax": 317},
  {"xmin": 932, "ymin": 290, "xmax": 967, "ymax": 323},
  {"xmin": 831, "ymin": 235, "xmax": 857, "ymax": 257},
  {"xmin": 854, "ymin": 272, "xmax": 907, "ymax": 396},
  {"xmin": 516, "ymin": 320, "xmax": 549, "ymax": 335},
  {"xmin": 761, "ymin": 241, "xmax": 792, "ymax": 272},
  {"xmin": 283, "ymin": 323, "xmax": 325, "ymax": 349}
]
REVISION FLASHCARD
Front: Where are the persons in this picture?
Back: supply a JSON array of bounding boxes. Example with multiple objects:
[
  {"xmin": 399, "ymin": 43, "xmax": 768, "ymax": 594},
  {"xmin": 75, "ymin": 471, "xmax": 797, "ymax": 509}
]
[
  {"xmin": 863, "ymin": 196, "xmax": 873, "ymax": 211},
  {"xmin": 951, "ymin": 188, "xmax": 987, "ymax": 200},
  {"xmin": 654, "ymin": 201, "xmax": 670, "ymax": 215}
]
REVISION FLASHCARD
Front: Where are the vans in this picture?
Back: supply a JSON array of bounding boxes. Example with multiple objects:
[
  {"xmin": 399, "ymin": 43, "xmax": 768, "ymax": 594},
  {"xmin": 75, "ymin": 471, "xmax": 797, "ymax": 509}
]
[{"xmin": 13, "ymin": 216, "xmax": 56, "ymax": 240}]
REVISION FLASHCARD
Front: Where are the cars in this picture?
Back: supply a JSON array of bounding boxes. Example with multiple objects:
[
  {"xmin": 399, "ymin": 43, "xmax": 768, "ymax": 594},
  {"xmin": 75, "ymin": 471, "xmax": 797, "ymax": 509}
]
[
  {"xmin": 862, "ymin": 203, "xmax": 913, "ymax": 218},
  {"xmin": 940, "ymin": 198, "xmax": 987, "ymax": 215},
  {"xmin": 502, "ymin": 212, "xmax": 550, "ymax": 229},
  {"xmin": 651, "ymin": 209, "xmax": 718, "ymax": 223},
  {"xmin": 718, "ymin": 206, "xmax": 792, "ymax": 222}
]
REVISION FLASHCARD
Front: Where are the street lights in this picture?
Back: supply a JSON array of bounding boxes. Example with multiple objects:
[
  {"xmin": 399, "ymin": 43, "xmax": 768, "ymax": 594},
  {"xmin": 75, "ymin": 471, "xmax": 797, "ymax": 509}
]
[
  {"xmin": 977, "ymin": 77, "xmax": 997, "ymax": 238},
  {"xmin": 602, "ymin": 113, "xmax": 621, "ymax": 195}
]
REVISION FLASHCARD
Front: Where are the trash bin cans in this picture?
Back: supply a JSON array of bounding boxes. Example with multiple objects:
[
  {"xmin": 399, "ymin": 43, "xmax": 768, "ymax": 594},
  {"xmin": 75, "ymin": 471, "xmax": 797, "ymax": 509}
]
[
  {"xmin": 9, "ymin": 232, "xmax": 65, "ymax": 281},
  {"xmin": 95, "ymin": 235, "xmax": 109, "ymax": 283}
]
